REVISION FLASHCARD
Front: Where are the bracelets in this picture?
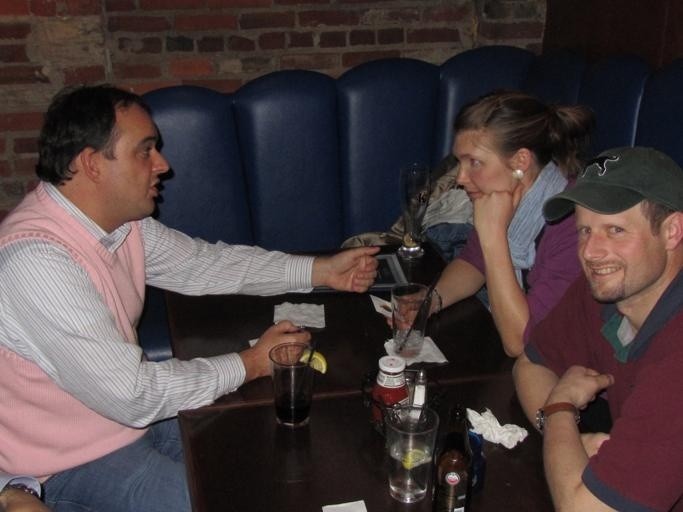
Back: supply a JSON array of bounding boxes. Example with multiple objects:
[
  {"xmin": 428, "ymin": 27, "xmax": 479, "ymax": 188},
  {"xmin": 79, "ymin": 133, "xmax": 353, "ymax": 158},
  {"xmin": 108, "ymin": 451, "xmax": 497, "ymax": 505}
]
[
  {"xmin": 4, "ymin": 483, "xmax": 41, "ymax": 499},
  {"xmin": 428, "ymin": 285, "xmax": 443, "ymax": 313}
]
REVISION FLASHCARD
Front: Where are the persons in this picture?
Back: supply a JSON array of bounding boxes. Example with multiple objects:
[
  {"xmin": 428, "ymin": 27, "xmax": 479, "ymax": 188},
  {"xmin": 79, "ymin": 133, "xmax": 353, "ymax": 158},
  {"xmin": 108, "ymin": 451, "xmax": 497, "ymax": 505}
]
[
  {"xmin": 388, "ymin": 89, "xmax": 586, "ymax": 360},
  {"xmin": 511, "ymin": 148, "xmax": 682, "ymax": 512},
  {"xmin": 0, "ymin": 86, "xmax": 382, "ymax": 512},
  {"xmin": 0, "ymin": 471, "xmax": 53, "ymax": 512}
]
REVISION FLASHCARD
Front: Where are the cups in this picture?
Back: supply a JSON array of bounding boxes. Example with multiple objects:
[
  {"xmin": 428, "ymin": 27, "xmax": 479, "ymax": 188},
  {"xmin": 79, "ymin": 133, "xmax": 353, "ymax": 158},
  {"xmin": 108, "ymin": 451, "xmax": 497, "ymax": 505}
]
[
  {"xmin": 397, "ymin": 163, "xmax": 430, "ymax": 259},
  {"xmin": 384, "ymin": 404, "xmax": 439, "ymax": 503},
  {"xmin": 267, "ymin": 342, "xmax": 311, "ymax": 428},
  {"xmin": 390, "ymin": 283, "xmax": 429, "ymax": 357}
]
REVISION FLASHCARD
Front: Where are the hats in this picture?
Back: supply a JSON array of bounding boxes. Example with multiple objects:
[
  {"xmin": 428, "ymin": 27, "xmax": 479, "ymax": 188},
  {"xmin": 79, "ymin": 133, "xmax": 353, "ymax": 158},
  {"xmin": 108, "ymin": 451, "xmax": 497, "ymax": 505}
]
[{"xmin": 541, "ymin": 145, "xmax": 682, "ymax": 226}]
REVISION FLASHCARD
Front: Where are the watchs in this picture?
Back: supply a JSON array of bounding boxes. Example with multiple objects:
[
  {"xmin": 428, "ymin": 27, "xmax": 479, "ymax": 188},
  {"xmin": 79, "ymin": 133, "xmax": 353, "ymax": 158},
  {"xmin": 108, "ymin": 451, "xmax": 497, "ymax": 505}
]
[{"xmin": 535, "ymin": 401, "xmax": 581, "ymax": 434}]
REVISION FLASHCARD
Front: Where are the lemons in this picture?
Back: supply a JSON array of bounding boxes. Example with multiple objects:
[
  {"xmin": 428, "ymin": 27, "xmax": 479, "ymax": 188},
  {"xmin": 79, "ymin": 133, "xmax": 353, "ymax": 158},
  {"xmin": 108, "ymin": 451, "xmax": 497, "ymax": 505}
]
[
  {"xmin": 403, "ymin": 449, "xmax": 428, "ymax": 469},
  {"xmin": 299, "ymin": 351, "xmax": 328, "ymax": 375}
]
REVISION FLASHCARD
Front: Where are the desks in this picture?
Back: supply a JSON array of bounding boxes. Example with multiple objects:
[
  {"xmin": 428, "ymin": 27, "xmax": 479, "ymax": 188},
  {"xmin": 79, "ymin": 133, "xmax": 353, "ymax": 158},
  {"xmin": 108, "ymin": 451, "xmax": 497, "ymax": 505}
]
[
  {"xmin": 158, "ymin": 286, "xmax": 522, "ymax": 406},
  {"xmin": 168, "ymin": 376, "xmax": 610, "ymax": 512}
]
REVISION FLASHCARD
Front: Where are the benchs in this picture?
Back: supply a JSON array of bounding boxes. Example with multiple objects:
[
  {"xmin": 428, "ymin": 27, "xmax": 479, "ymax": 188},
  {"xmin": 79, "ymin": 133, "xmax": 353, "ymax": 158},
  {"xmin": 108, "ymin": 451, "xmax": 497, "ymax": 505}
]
[{"xmin": 129, "ymin": 43, "xmax": 681, "ymax": 363}]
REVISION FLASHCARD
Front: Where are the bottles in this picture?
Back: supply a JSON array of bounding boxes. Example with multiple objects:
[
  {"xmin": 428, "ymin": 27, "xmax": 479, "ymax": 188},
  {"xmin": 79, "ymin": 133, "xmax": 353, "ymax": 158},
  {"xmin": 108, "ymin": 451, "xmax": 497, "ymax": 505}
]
[
  {"xmin": 413, "ymin": 368, "xmax": 428, "ymax": 408},
  {"xmin": 469, "ymin": 431, "xmax": 485, "ymax": 492},
  {"xmin": 371, "ymin": 355, "xmax": 409, "ymax": 428},
  {"xmin": 430, "ymin": 404, "xmax": 471, "ymax": 512},
  {"xmin": 404, "ymin": 375, "xmax": 413, "ymax": 405}
]
